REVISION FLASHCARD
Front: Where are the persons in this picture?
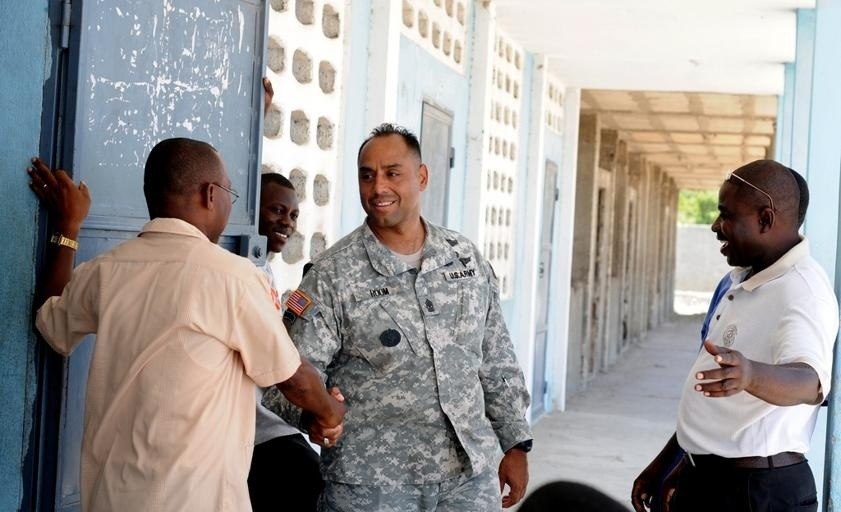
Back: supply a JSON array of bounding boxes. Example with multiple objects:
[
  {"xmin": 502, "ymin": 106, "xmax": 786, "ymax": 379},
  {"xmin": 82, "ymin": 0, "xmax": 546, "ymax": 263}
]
[
  {"xmin": 630, "ymin": 158, "xmax": 840, "ymax": 512},
  {"xmin": 248, "ymin": 77, "xmax": 322, "ymax": 512},
  {"xmin": 261, "ymin": 123, "xmax": 535, "ymax": 511},
  {"xmin": 26, "ymin": 139, "xmax": 346, "ymax": 511}
]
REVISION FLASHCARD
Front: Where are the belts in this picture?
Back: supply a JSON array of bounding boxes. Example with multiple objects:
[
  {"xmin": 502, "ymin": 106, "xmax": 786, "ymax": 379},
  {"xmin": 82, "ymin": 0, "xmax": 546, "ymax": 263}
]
[{"xmin": 681, "ymin": 451, "xmax": 806, "ymax": 471}]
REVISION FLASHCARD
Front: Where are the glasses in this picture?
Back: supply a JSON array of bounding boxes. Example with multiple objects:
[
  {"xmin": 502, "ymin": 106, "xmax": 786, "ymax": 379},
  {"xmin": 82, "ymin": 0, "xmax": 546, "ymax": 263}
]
[
  {"xmin": 213, "ymin": 182, "xmax": 240, "ymax": 205},
  {"xmin": 725, "ymin": 170, "xmax": 774, "ymax": 209}
]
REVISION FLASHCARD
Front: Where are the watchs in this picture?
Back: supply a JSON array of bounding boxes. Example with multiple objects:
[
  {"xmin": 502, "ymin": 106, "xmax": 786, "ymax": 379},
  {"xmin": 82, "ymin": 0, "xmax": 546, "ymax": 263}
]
[
  {"xmin": 46, "ymin": 232, "xmax": 78, "ymax": 252},
  {"xmin": 511, "ymin": 435, "xmax": 534, "ymax": 454}
]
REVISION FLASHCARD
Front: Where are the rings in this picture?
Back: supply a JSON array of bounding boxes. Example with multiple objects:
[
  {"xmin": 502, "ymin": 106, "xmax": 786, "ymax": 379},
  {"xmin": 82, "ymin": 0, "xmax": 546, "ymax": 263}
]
[{"xmin": 324, "ymin": 438, "xmax": 330, "ymax": 446}]
